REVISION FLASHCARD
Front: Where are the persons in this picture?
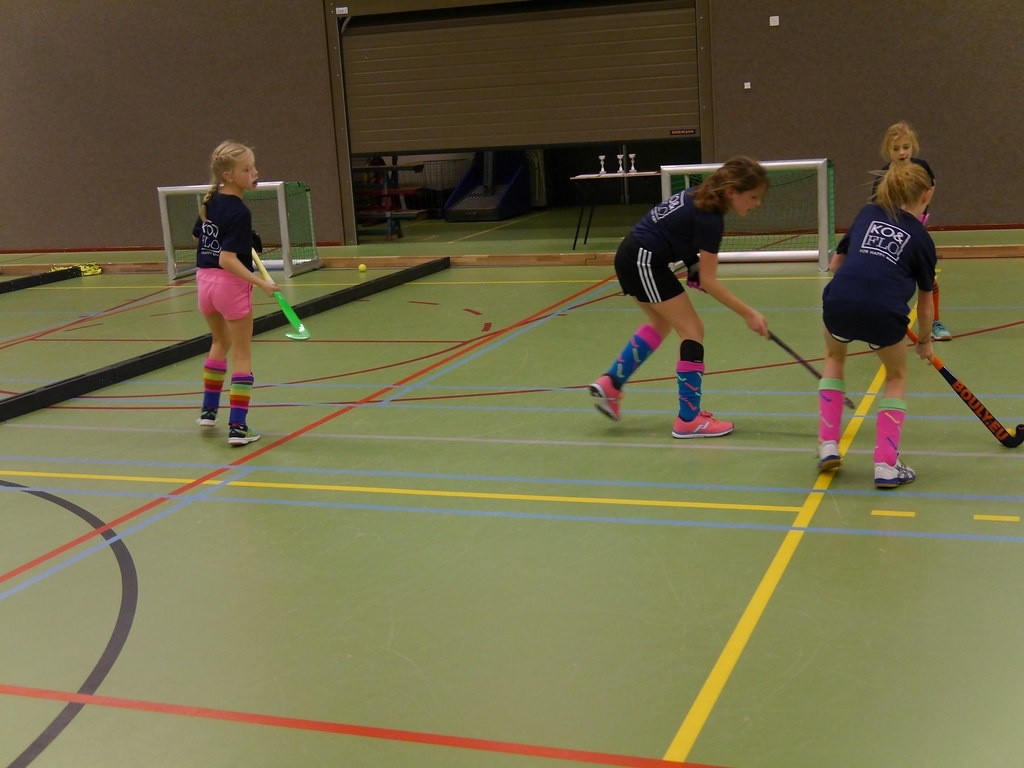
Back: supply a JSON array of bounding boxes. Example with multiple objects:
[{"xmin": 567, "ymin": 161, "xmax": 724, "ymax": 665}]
[
  {"xmin": 589, "ymin": 157, "xmax": 772, "ymax": 438},
  {"xmin": 873, "ymin": 124, "xmax": 953, "ymax": 340},
  {"xmin": 817, "ymin": 163, "xmax": 934, "ymax": 488},
  {"xmin": 192, "ymin": 143, "xmax": 280, "ymax": 445}
]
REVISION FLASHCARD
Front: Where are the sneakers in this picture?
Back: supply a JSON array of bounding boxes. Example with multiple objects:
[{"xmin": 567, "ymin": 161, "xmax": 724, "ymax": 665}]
[
  {"xmin": 671, "ymin": 409, "xmax": 734, "ymax": 438},
  {"xmin": 874, "ymin": 452, "xmax": 916, "ymax": 487},
  {"xmin": 931, "ymin": 320, "xmax": 951, "ymax": 340},
  {"xmin": 228, "ymin": 424, "xmax": 260, "ymax": 445},
  {"xmin": 590, "ymin": 374, "xmax": 622, "ymax": 423},
  {"xmin": 816, "ymin": 439, "xmax": 842, "ymax": 470},
  {"xmin": 196, "ymin": 408, "xmax": 220, "ymax": 427}
]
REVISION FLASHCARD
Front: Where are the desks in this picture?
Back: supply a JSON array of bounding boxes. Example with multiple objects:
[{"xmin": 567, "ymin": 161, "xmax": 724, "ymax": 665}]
[{"xmin": 569, "ymin": 170, "xmax": 662, "ymax": 251}]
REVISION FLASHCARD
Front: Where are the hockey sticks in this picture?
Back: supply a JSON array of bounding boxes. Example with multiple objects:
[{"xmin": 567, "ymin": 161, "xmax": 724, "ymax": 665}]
[
  {"xmin": 253, "ymin": 246, "xmax": 311, "ymax": 341},
  {"xmin": 769, "ymin": 329, "xmax": 857, "ymax": 410},
  {"xmin": 906, "ymin": 328, "xmax": 1024, "ymax": 448}
]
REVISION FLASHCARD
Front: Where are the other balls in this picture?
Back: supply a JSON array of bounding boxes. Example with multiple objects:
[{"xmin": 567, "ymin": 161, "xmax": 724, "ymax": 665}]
[
  {"xmin": 358, "ymin": 263, "xmax": 367, "ymax": 272},
  {"xmin": 1006, "ymin": 427, "xmax": 1015, "ymax": 436}
]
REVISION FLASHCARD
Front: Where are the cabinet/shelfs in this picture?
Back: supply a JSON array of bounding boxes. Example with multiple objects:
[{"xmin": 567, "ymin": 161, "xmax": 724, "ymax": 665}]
[{"xmin": 352, "ymin": 162, "xmax": 425, "ymax": 240}]
